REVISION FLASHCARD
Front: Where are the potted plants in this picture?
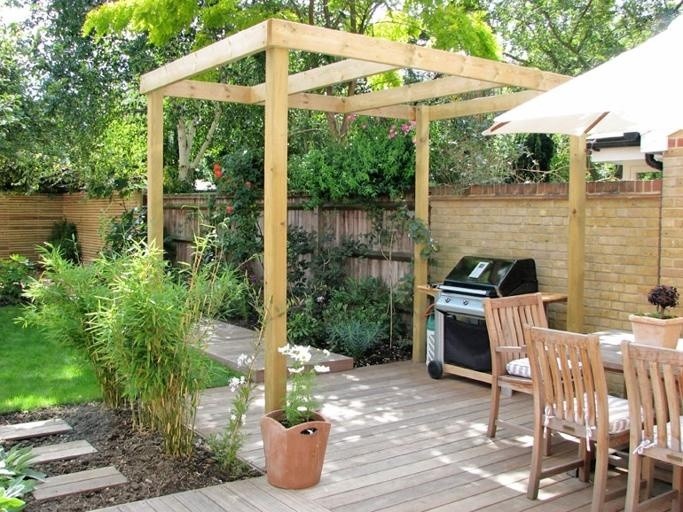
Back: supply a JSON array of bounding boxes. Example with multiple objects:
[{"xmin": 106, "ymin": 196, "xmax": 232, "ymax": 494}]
[{"xmin": 628, "ymin": 283, "xmax": 683, "ymax": 351}]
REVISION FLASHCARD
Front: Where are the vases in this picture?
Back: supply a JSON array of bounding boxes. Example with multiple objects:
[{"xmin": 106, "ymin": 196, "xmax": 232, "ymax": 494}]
[{"xmin": 260, "ymin": 409, "xmax": 331, "ymax": 489}]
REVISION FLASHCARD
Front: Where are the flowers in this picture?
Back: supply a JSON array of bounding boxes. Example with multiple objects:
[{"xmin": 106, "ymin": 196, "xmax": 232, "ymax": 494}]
[{"xmin": 278, "ymin": 343, "xmax": 331, "ymax": 426}]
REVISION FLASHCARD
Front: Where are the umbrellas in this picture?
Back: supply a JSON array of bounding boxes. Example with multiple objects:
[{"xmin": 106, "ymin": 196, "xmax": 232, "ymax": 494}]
[{"xmin": 480, "ymin": 9, "xmax": 683, "ymax": 156}]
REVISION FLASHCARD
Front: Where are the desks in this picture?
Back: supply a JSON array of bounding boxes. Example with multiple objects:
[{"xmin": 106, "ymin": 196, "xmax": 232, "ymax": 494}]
[{"xmin": 566, "ymin": 328, "xmax": 683, "ymax": 384}]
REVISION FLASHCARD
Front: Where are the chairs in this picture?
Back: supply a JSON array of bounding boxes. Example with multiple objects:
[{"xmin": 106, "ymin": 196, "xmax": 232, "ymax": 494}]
[
  {"xmin": 480, "ymin": 292, "xmax": 585, "ymax": 457},
  {"xmin": 620, "ymin": 340, "xmax": 683, "ymax": 511},
  {"xmin": 522, "ymin": 323, "xmax": 669, "ymax": 511}
]
